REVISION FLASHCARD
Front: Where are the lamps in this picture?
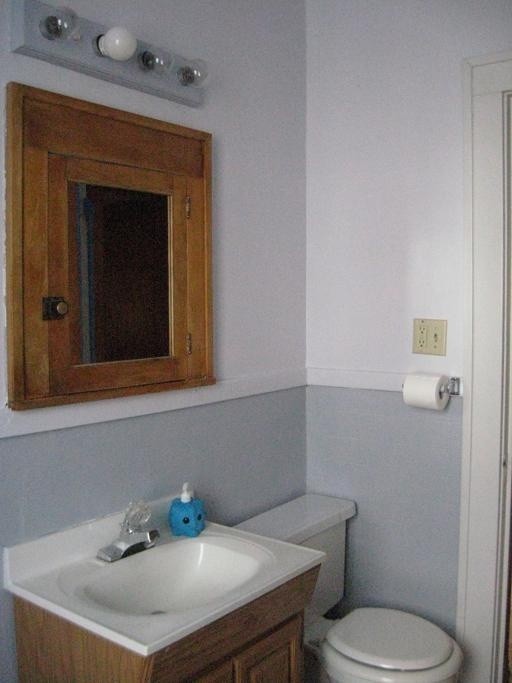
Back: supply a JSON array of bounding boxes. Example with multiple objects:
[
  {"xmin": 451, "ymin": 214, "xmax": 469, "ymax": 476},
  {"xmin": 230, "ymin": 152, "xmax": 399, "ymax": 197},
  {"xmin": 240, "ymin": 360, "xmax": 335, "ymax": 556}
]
[{"xmin": 11, "ymin": 0, "xmax": 208, "ymax": 106}]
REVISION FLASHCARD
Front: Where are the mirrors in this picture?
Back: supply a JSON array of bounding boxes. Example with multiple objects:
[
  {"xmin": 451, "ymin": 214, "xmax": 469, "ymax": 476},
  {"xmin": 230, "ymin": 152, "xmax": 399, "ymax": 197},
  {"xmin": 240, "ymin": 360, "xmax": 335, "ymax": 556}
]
[{"xmin": 47, "ymin": 153, "xmax": 185, "ymax": 395}]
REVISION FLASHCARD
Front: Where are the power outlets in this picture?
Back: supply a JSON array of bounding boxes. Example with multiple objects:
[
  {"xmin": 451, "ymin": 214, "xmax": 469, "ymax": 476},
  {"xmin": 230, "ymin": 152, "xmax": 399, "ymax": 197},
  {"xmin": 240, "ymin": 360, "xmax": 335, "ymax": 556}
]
[{"xmin": 413, "ymin": 319, "xmax": 447, "ymax": 355}]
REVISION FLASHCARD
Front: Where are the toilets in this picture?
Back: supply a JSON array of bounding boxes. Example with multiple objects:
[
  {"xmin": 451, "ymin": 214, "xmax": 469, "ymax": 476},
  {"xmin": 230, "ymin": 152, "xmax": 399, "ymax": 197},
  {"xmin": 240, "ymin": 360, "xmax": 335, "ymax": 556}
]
[{"xmin": 231, "ymin": 494, "xmax": 464, "ymax": 683}]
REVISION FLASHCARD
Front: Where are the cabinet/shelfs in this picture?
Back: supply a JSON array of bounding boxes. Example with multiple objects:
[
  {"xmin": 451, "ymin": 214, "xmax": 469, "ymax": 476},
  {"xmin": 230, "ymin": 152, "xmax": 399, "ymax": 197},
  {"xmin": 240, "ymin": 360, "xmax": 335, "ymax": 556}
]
[{"xmin": 138, "ymin": 564, "xmax": 320, "ymax": 683}]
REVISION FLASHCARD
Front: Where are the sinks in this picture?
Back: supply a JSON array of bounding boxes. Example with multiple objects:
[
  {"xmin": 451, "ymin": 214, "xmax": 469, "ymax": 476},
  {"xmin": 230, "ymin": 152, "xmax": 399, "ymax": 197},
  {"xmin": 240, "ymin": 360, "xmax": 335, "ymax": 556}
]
[{"xmin": 13, "ymin": 520, "xmax": 326, "ymax": 657}]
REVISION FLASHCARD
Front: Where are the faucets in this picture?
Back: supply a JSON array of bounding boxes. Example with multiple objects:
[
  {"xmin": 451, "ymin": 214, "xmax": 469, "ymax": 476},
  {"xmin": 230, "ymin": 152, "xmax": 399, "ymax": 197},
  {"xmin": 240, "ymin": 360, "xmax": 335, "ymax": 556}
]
[{"xmin": 96, "ymin": 501, "xmax": 159, "ymax": 562}]
